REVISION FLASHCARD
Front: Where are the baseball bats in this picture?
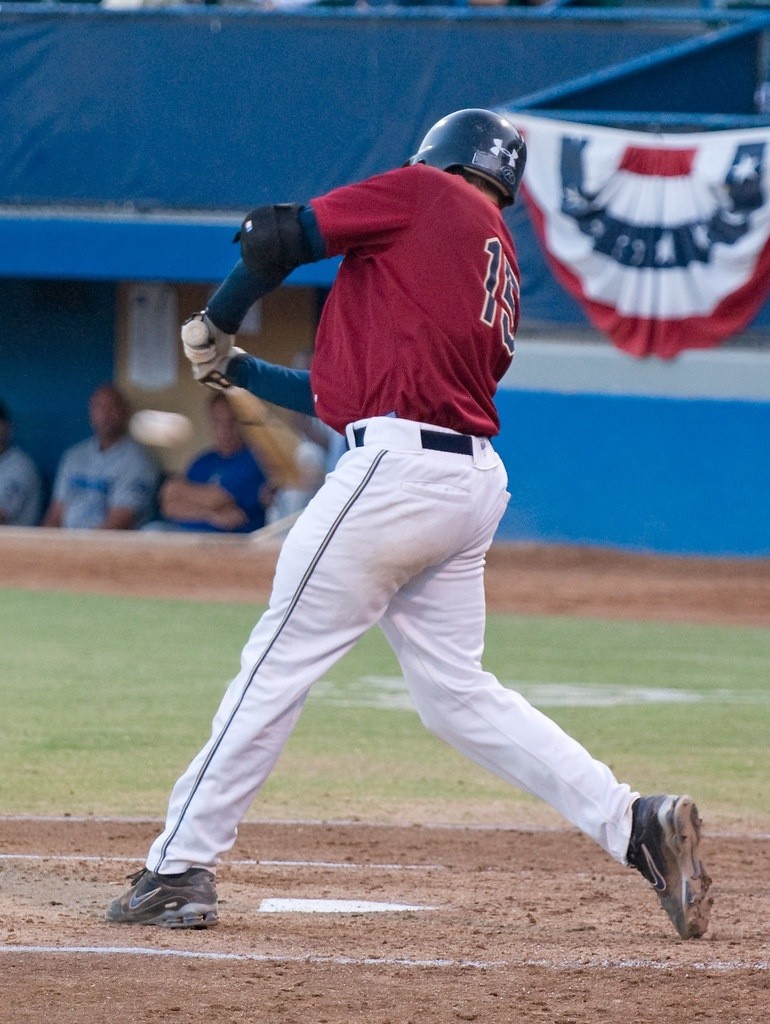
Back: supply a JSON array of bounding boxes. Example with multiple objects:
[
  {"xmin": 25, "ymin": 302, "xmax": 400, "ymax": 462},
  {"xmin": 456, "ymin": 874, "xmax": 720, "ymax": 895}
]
[{"xmin": 186, "ymin": 321, "xmax": 324, "ymax": 475}]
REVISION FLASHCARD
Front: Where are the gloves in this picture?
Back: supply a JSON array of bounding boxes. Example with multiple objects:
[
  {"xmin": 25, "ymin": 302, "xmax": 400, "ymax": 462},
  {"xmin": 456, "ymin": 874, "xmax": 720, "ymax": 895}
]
[
  {"xmin": 180, "ymin": 313, "xmax": 235, "ymax": 364},
  {"xmin": 193, "ymin": 346, "xmax": 245, "ymax": 389}
]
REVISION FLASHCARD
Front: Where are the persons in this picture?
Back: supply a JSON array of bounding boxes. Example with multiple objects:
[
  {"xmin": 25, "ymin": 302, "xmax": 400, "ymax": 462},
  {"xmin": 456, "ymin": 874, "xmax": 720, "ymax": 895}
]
[
  {"xmin": 107, "ymin": 110, "xmax": 715, "ymax": 941},
  {"xmin": 0, "ymin": 386, "xmax": 346, "ymax": 536}
]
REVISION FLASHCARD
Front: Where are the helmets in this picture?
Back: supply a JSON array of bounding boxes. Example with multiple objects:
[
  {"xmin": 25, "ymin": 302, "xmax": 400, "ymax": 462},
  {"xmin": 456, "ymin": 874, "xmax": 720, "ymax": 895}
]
[{"xmin": 398, "ymin": 107, "xmax": 528, "ymax": 206}]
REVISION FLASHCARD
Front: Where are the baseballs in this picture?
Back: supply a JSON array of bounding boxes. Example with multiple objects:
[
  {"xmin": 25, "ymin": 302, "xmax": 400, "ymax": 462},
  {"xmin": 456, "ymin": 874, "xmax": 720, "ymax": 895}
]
[{"xmin": 130, "ymin": 408, "xmax": 186, "ymax": 448}]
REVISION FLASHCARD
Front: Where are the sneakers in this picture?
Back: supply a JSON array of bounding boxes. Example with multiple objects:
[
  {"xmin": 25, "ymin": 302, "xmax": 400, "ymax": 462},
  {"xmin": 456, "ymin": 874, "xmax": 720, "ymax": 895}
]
[
  {"xmin": 625, "ymin": 794, "xmax": 714, "ymax": 942},
  {"xmin": 105, "ymin": 869, "xmax": 220, "ymax": 928}
]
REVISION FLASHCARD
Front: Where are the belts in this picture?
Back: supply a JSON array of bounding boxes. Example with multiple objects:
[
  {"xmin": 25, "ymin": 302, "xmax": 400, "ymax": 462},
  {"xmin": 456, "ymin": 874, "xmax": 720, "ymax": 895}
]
[{"xmin": 344, "ymin": 426, "xmax": 471, "ymax": 455}]
